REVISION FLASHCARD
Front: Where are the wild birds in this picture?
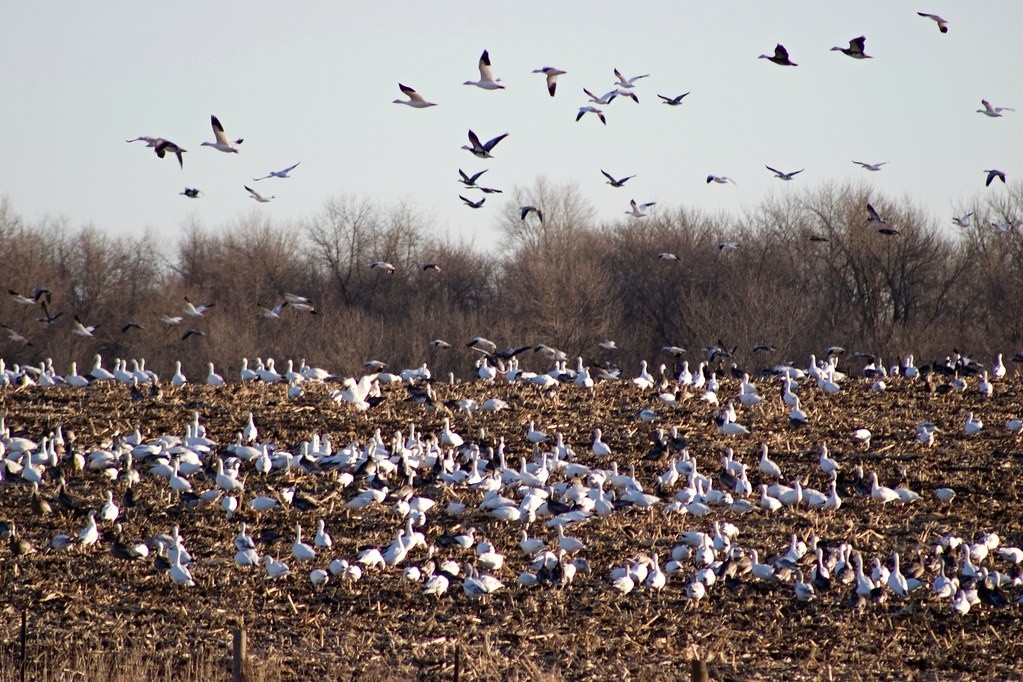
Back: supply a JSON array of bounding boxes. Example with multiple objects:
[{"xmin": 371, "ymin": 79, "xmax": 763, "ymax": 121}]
[{"xmin": 0, "ymin": 13, "xmax": 1023, "ymax": 617}]
